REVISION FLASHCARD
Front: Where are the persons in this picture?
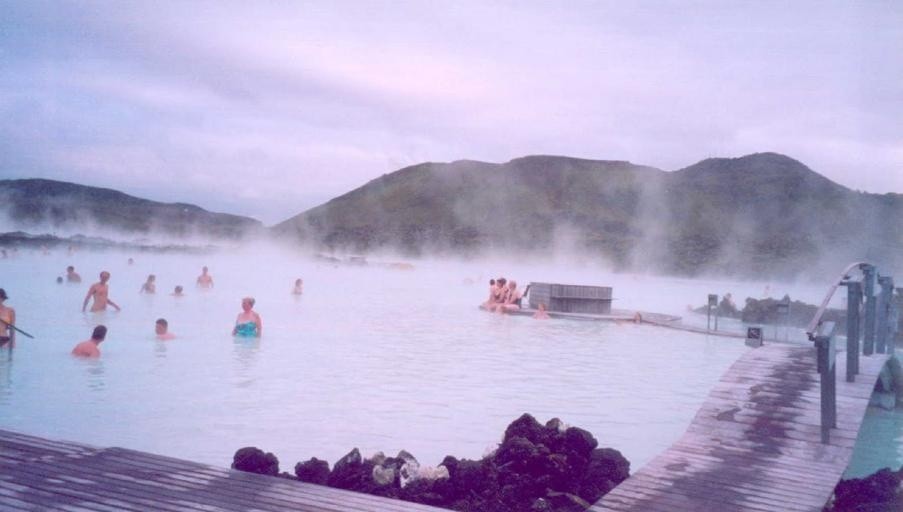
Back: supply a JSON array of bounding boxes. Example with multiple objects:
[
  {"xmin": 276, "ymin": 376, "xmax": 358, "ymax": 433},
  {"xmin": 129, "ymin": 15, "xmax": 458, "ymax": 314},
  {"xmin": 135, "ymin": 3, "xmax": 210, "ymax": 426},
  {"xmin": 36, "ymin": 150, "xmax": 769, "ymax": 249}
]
[
  {"xmin": 56, "ymin": 276, "xmax": 63, "ymax": 284},
  {"xmin": 290, "ymin": 278, "xmax": 304, "ymax": 294},
  {"xmin": 168, "ymin": 285, "xmax": 184, "ymax": 296},
  {"xmin": 532, "ymin": 303, "xmax": 552, "ymax": 319},
  {"xmin": 724, "ymin": 293, "xmax": 733, "ymax": 307},
  {"xmin": 81, "ymin": 271, "xmax": 120, "ymax": 314},
  {"xmin": 633, "ymin": 312, "xmax": 642, "ymax": 325},
  {"xmin": 138, "ymin": 274, "xmax": 156, "ymax": 294},
  {"xmin": 70, "ymin": 324, "xmax": 107, "ymax": 360},
  {"xmin": 482, "ymin": 277, "xmax": 522, "ymax": 317},
  {"xmin": 231, "ymin": 295, "xmax": 263, "ymax": 340},
  {"xmin": 0, "ymin": 288, "xmax": 16, "ymax": 352},
  {"xmin": 153, "ymin": 318, "xmax": 178, "ymax": 339},
  {"xmin": 65, "ymin": 266, "xmax": 80, "ymax": 282},
  {"xmin": 195, "ymin": 266, "xmax": 215, "ymax": 288}
]
